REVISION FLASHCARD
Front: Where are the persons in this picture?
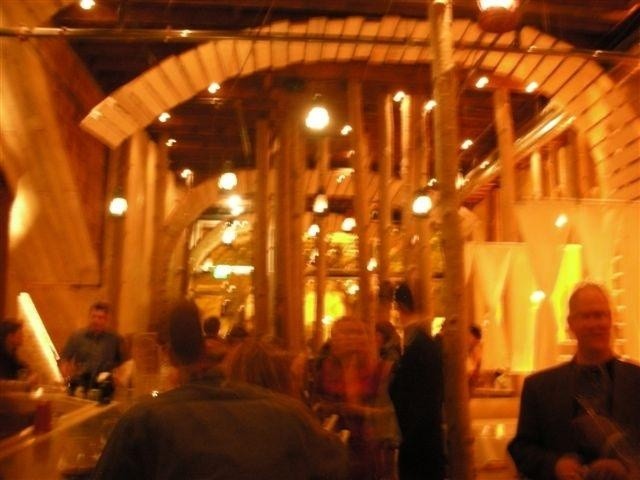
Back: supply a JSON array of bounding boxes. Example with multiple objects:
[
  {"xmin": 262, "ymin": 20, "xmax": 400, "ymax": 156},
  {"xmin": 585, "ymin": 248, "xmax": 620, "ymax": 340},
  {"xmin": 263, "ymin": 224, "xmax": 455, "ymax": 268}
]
[
  {"xmin": 129, "ymin": 279, "xmax": 483, "ymax": 480},
  {"xmin": 0, "ymin": 316, "xmax": 35, "ymax": 380},
  {"xmin": 502, "ymin": 280, "xmax": 639, "ymax": 478},
  {"xmin": 56, "ymin": 301, "xmax": 127, "ymax": 409},
  {"xmin": 89, "ymin": 290, "xmax": 355, "ymax": 479}
]
